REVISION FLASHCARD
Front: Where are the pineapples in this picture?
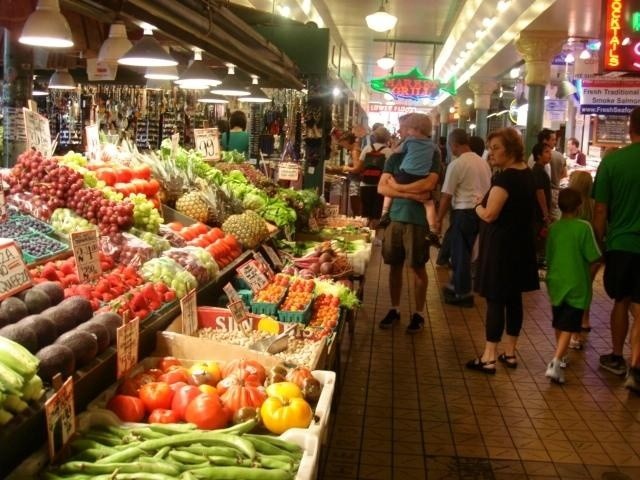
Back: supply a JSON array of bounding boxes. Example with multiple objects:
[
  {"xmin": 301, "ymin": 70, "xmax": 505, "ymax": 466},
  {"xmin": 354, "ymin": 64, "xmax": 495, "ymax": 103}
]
[{"xmin": 143, "ymin": 149, "xmax": 269, "ymax": 250}]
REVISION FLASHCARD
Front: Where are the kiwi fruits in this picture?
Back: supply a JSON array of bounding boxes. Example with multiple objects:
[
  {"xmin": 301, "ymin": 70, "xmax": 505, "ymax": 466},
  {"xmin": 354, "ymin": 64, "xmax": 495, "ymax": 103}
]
[{"xmin": 0, "ymin": 279, "xmax": 125, "ymax": 386}]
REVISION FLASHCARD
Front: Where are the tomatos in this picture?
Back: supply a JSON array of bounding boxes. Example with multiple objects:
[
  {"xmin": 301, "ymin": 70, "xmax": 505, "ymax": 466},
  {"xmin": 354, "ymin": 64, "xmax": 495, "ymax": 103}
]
[
  {"xmin": 108, "ymin": 354, "xmax": 322, "ymax": 435},
  {"xmin": 87, "ymin": 155, "xmax": 244, "ymax": 269}
]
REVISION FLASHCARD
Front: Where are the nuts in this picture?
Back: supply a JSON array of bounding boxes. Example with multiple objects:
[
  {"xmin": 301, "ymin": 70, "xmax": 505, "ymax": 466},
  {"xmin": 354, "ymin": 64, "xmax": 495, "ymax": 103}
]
[{"xmin": 191, "ymin": 327, "xmax": 321, "ymax": 363}]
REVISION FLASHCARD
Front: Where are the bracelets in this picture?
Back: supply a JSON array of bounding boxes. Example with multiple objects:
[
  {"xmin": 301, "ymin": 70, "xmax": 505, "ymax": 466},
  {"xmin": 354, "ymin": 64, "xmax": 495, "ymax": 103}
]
[{"xmin": 475, "ymin": 203, "xmax": 482, "ymax": 210}]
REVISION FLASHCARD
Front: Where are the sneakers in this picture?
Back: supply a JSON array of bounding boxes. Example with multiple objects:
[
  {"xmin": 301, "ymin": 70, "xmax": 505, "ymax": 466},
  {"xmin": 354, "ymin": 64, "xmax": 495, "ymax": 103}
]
[
  {"xmin": 377, "ymin": 215, "xmax": 389, "ymax": 227},
  {"xmin": 425, "ymin": 231, "xmax": 441, "ymax": 249},
  {"xmin": 380, "ymin": 261, "xmax": 640, "ymax": 389}
]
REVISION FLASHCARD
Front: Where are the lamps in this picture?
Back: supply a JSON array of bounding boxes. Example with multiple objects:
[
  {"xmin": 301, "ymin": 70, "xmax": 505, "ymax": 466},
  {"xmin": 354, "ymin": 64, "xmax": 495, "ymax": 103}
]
[
  {"xmin": 557, "ymin": 40, "xmax": 593, "ymax": 99},
  {"xmin": 18, "ymin": 0, "xmax": 273, "ymax": 104},
  {"xmin": 366, "ymin": 0, "xmax": 397, "ymax": 101}
]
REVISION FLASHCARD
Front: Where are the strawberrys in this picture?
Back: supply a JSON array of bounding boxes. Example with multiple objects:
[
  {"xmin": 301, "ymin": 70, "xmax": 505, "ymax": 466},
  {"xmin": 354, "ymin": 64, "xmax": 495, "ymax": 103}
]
[{"xmin": 27, "ymin": 247, "xmax": 175, "ymax": 324}]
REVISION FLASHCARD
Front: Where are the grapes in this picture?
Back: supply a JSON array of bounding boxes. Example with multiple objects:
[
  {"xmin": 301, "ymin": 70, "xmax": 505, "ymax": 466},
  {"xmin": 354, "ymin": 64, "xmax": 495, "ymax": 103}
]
[{"xmin": 3, "ymin": 147, "xmax": 218, "ymax": 300}]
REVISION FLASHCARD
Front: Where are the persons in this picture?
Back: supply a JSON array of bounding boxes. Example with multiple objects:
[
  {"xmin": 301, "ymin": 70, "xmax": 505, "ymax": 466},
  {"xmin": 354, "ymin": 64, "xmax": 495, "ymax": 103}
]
[
  {"xmin": 434, "ymin": 129, "xmax": 493, "ymax": 308},
  {"xmin": 566, "ymin": 137, "xmax": 587, "ymax": 168},
  {"xmin": 344, "ymin": 123, "xmax": 399, "ymax": 231},
  {"xmin": 379, "ymin": 113, "xmax": 441, "ymax": 247},
  {"xmin": 545, "ymin": 171, "xmax": 603, "ymax": 382},
  {"xmin": 377, "ymin": 114, "xmax": 442, "ymax": 332},
  {"xmin": 467, "ymin": 127, "xmax": 540, "ymax": 373},
  {"xmin": 220, "ymin": 111, "xmax": 249, "ymax": 160},
  {"xmin": 594, "ymin": 107, "xmax": 640, "ymax": 391},
  {"xmin": 527, "ymin": 129, "xmax": 567, "ymax": 267}
]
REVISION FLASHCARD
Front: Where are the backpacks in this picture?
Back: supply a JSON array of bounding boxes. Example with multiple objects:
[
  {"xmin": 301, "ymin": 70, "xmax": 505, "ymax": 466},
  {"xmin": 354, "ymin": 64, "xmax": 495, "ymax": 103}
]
[{"xmin": 361, "ymin": 144, "xmax": 387, "ymax": 183}]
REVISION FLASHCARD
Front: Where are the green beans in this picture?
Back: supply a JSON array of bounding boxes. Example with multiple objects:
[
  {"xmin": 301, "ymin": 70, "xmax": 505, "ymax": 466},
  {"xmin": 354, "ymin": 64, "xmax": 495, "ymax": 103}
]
[{"xmin": 44, "ymin": 424, "xmax": 302, "ymax": 480}]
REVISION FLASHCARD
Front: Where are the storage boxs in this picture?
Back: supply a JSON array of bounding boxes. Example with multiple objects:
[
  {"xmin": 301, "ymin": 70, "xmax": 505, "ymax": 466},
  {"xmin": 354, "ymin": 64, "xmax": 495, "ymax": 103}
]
[{"xmin": 155, "ymin": 306, "xmax": 327, "ymax": 372}]
